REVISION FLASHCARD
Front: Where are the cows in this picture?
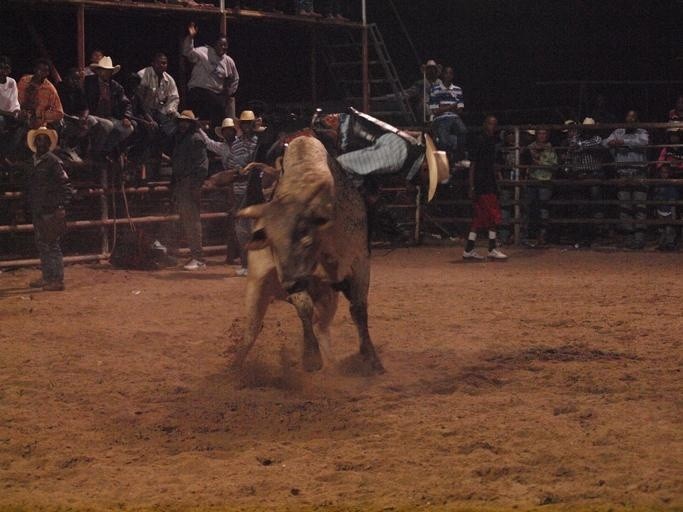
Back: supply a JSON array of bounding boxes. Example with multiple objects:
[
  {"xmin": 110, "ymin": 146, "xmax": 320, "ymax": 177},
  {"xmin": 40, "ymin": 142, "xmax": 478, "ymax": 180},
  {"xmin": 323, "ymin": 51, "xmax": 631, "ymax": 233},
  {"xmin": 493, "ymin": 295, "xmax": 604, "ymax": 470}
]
[{"xmin": 224, "ymin": 134, "xmax": 389, "ymax": 377}]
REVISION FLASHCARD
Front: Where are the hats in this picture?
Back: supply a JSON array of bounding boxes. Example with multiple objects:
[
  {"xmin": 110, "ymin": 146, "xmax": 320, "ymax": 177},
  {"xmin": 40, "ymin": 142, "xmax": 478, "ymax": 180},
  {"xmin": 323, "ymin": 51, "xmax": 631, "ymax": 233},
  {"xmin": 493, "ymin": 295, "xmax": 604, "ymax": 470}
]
[
  {"xmin": 421, "ymin": 131, "xmax": 451, "ymax": 203},
  {"xmin": 214, "ymin": 117, "xmax": 242, "ymax": 139},
  {"xmin": 177, "ymin": 110, "xmax": 198, "ymax": 122},
  {"xmin": 26, "ymin": 125, "xmax": 59, "ymax": 154},
  {"xmin": 89, "ymin": 56, "xmax": 121, "ymax": 76},
  {"xmin": 575, "ymin": 117, "xmax": 600, "ymax": 130},
  {"xmin": 234, "ymin": 110, "xmax": 262, "ymax": 129},
  {"xmin": 420, "ymin": 60, "xmax": 442, "ymax": 77}
]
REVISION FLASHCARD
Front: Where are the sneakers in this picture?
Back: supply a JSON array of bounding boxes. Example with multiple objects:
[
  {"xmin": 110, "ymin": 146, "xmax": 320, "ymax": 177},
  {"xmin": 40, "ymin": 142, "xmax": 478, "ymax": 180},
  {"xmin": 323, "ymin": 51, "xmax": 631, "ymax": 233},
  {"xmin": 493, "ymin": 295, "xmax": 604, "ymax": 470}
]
[
  {"xmin": 183, "ymin": 258, "xmax": 206, "ymax": 272},
  {"xmin": 29, "ymin": 278, "xmax": 48, "ymax": 289},
  {"xmin": 234, "ymin": 267, "xmax": 248, "ymax": 276},
  {"xmin": 486, "ymin": 248, "xmax": 506, "ymax": 258},
  {"xmin": 462, "ymin": 249, "xmax": 484, "ymax": 259},
  {"xmin": 42, "ymin": 281, "xmax": 65, "ymax": 292}
]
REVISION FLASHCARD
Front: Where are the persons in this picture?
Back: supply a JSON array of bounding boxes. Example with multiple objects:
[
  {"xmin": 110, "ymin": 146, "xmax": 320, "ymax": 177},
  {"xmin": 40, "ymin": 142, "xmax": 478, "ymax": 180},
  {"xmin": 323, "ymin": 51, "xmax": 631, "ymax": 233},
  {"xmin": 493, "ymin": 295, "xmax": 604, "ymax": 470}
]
[
  {"xmin": 1, "ymin": 19, "xmax": 263, "ymax": 291},
  {"xmin": 386, "ymin": 61, "xmax": 683, "ymax": 260}
]
